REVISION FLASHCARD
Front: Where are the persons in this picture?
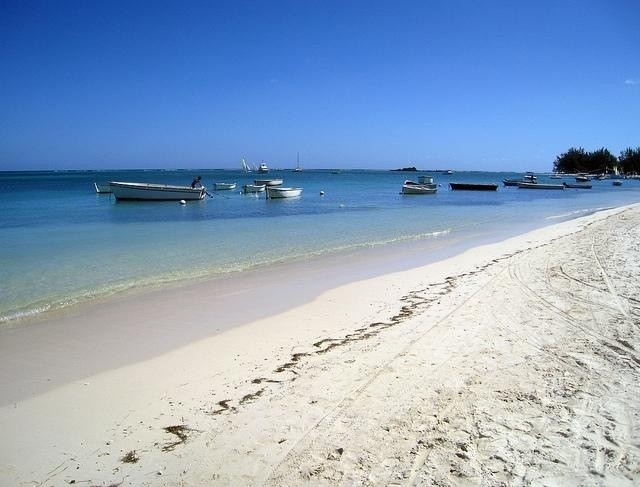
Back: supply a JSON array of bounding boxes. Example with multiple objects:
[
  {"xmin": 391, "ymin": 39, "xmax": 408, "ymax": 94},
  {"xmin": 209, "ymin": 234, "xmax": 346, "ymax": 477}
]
[
  {"xmin": 530, "ymin": 174, "xmax": 535, "ymax": 183},
  {"xmin": 191, "ymin": 176, "xmax": 202, "ymax": 188}
]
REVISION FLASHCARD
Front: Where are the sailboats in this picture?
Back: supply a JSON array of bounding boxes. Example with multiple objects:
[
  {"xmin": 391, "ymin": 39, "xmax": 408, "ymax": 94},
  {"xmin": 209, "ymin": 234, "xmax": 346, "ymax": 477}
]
[
  {"xmin": 240, "ymin": 154, "xmax": 253, "ymax": 174},
  {"xmin": 295, "ymin": 152, "xmax": 303, "ymax": 172}
]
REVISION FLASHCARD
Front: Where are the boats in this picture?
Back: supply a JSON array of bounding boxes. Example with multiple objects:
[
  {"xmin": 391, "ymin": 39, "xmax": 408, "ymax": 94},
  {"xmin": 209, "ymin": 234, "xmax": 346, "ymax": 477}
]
[
  {"xmin": 599, "ymin": 174, "xmax": 605, "ymax": 180},
  {"xmin": 526, "ymin": 171, "xmax": 535, "ymax": 174},
  {"xmin": 330, "ymin": 170, "xmax": 341, "ymax": 175},
  {"xmin": 519, "ymin": 176, "xmax": 565, "ymax": 190},
  {"xmin": 243, "ymin": 184, "xmax": 266, "ymax": 193},
  {"xmin": 214, "ymin": 181, "xmax": 237, "ymax": 191},
  {"xmin": 447, "ymin": 169, "xmax": 453, "ymax": 174},
  {"xmin": 264, "ymin": 185, "xmax": 304, "ymax": 200},
  {"xmin": 401, "ymin": 175, "xmax": 437, "ymax": 195},
  {"xmin": 92, "ymin": 181, "xmax": 113, "ymax": 195},
  {"xmin": 576, "ymin": 174, "xmax": 591, "ymax": 182},
  {"xmin": 503, "ymin": 176, "xmax": 538, "ymax": 186},
  {"xmin": 259, "ymin": 160, "xmax": 269, "ymax": 173},
  {"xmin": 111, "ymin": 179, "xmax": 208, "ymax": 202},
  {"xmin": 254, "ymin": 178, "xmax": 284, "ymax": 186},
  {"xmin": 550, "ymin": 176, "xmax": 562, "ymax": 179},
  {"xmin": 605, "ymin": 162, "xmax": 640, "ymax": 180},
  {"xmin": 612, "ymin": 180, "xmax": 623, "ymax": 186},
  {"xmin": 449, "ymin": 182, "xmax": 498, "ymax": 191},
  {"xmin": 563, "ymin": 181, "xmax": 593, "ymax": 189}
]
